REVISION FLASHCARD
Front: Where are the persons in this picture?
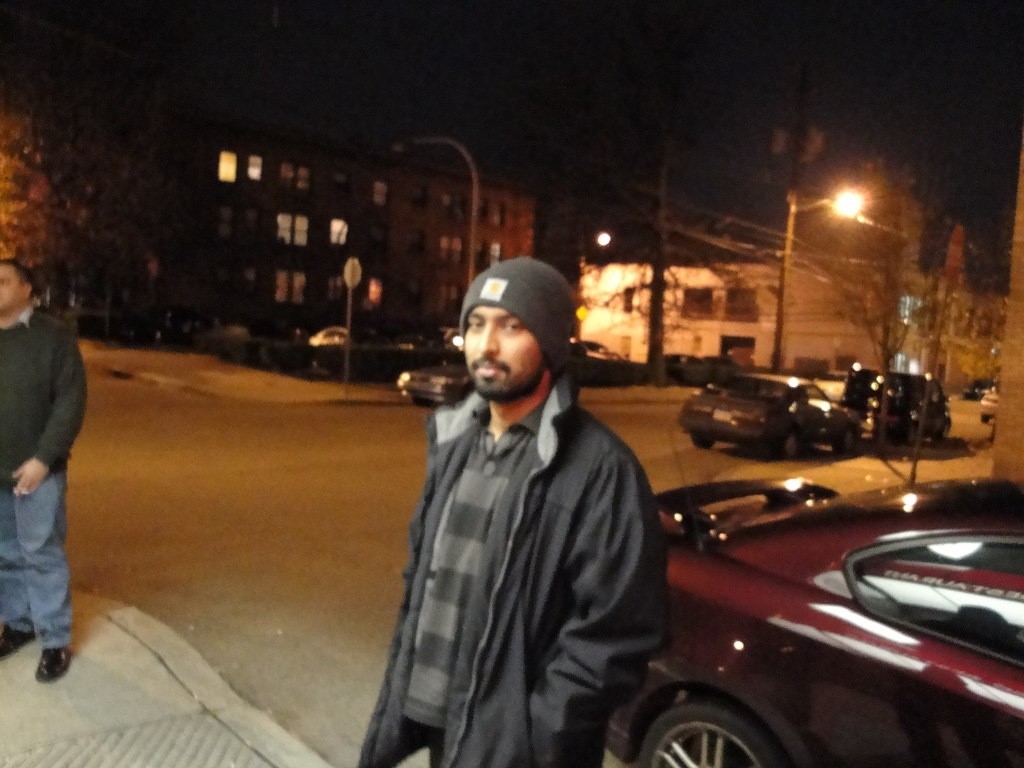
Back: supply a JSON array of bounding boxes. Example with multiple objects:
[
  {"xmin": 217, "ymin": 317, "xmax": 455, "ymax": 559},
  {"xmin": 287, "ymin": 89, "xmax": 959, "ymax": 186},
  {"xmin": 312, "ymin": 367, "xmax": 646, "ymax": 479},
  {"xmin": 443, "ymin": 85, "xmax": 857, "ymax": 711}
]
[
  {"xmin": 0, "ymin": 259, "xmax": 91, "ymax": 685},
  {"xmin": 354, "ymin": 257, "xmax": 673, "ymax": 768}
]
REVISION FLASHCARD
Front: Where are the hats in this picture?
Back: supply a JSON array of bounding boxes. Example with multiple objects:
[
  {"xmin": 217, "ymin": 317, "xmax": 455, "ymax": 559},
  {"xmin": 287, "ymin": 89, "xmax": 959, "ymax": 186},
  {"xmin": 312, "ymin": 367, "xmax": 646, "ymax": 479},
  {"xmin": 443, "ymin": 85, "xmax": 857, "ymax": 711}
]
[{"xmin": 459, "ymin": 258, "xmax": 575, "ymax": 369}]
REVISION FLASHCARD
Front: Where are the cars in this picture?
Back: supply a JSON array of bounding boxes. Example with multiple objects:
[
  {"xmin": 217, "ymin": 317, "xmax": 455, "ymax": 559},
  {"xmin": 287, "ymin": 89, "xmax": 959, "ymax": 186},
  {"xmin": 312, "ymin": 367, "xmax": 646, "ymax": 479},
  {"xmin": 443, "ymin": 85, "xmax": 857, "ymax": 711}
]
[
  {"xmin": 576, "ymin": 338, "xmax": 621, "ymax": 361},
  {"xmin": 679, "ymin": 374, "xmax": 863, "ymax": 456},
  {"xmin": 606, "ymin": 475, "xmax": 1024, "ymax": 768},
  {"xmin": 294, "ymin": 325, "xmax": 348, "ymax": 347},
  {"xmin": 397, "ymin": 362, "xmax": 474, "ymax": 408},
  {"xmin": 979, "ymin": 388, "xmax": 998, "ymax": 420}
]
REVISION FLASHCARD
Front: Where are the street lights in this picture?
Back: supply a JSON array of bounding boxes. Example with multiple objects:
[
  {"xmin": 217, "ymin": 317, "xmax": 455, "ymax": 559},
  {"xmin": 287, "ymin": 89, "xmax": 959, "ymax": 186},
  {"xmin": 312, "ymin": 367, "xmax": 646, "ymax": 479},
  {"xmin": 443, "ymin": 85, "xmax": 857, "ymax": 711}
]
[
  {"xmin": 387, "ymin": 136, "xmax": 479, "ymax": 304},
  {"xmin": 769, "ymin": 192, "xmax": 864, "ymax": 372}
]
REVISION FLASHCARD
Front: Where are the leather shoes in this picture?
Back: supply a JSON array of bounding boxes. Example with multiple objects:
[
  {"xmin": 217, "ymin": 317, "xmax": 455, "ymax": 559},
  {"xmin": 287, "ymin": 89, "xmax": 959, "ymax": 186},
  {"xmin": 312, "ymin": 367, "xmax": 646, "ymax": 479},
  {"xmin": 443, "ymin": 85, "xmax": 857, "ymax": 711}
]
[
  {"xmin": 38, "ymin": 647, "xmax": 70, "ymax": 682},
  {"xmin": 0, "ymin": 626, "xmax": 35, "ymax": 659}
]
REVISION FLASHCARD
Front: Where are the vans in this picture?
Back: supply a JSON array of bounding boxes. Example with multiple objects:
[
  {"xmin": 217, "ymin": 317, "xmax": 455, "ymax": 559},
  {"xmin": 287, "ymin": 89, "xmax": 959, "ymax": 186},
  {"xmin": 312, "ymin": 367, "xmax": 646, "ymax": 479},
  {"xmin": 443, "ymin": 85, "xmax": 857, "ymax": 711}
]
[{"xmin": 841, "ymin": 370, "xmax": 952, "ymax": 443}]
